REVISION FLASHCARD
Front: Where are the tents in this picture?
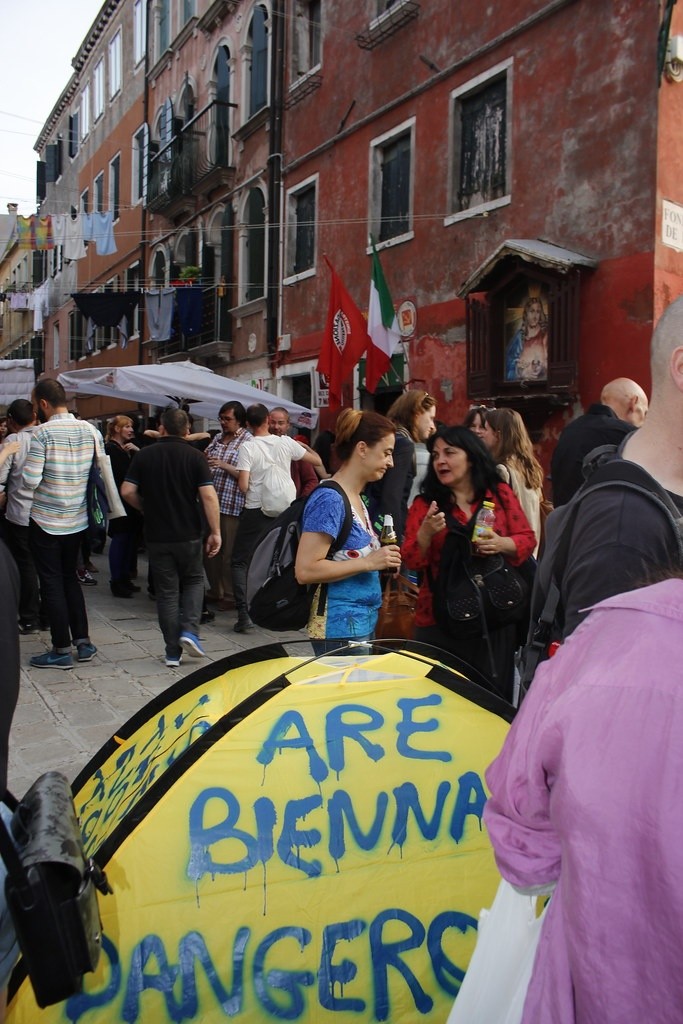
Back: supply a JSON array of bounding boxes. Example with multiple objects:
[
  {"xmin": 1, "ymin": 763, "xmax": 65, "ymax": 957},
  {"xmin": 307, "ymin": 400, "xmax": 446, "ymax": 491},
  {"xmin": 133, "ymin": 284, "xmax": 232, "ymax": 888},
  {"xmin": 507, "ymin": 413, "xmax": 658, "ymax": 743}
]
[{"xmin": 0, "ymin": 637, "xmax": 565, "ymax": 1024}]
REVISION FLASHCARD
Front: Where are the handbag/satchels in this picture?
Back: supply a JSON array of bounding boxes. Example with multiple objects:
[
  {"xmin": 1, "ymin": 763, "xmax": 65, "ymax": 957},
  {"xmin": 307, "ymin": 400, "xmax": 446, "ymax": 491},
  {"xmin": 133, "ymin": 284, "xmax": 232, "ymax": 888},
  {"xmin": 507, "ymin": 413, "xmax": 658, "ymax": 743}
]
[
  {"xmin": 445, "ymin": 878, "xmax": 551, "ymax": 1024},
  {"xmin": 85, "ymin": 421, "xmax": 128, "ymax": 519},
  {"xmin": 86, "ymin": 464, "xmax": 108, "ymax": 555},
  {"xmin": 6, "ymin": 774, "xmax": 114, "ymax": 1008},
  {"xmin": 376, "ymin": 573, "xmax": 418, "ymax": 639}
]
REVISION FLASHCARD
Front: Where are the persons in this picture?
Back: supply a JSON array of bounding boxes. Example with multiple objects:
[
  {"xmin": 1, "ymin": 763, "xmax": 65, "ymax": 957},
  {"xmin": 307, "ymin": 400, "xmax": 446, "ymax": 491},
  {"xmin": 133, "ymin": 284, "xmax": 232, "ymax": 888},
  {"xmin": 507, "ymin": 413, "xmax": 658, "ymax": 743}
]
[
  {"xmin": 363, "ymin": 390, "xmax": 438, "ymax": 598},
  {"xmin": 460, "ymin": 405, "xmax": 498, "ymax": 461},
  {"xmin": 22, "ymin": 378, "xmax": 108, "ymax": 670},
  {"xmin": 0, "ymin": 399, "xmax": 448, "ymax": 635},
  {"xmin": 0, "ymin": 543, "xmax": 20, "ymax": 985},
  {"xmin": 519, "ymin": 295, "xmax": 683, "ymax": 711},
  {"xmin": 434, "ymin": 419, "xmax": 445, "ymax": 433},
  {"xmin": 399, "ymin": 425, "xmax": 537, "ymax": 692},
  {"xmin": 482, "ymin": 572, "xmax": 683, "ymax": 1024},
  {"xmin": 121, "ymin": 409, "xmax": 222, "ymax": 667},
  {"xmin": 550, "ymin": 377, "xmax": 649, "ymax": 512},
  {"xmin": 482, "ymin": 408, "xmax": 544, "ymax": 660},
  {"xmin": 295, "ymin": 407, "xmax": 402, "ymax": 656}
]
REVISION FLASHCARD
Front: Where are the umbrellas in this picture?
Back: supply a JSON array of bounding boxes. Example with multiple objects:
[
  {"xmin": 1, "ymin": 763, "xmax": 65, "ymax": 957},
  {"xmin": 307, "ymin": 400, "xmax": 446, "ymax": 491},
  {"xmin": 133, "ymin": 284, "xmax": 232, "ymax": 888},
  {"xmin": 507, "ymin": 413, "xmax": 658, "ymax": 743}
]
[{"xmin": 58, "ymin": 361, "xmax": 319, "ymax": 431}]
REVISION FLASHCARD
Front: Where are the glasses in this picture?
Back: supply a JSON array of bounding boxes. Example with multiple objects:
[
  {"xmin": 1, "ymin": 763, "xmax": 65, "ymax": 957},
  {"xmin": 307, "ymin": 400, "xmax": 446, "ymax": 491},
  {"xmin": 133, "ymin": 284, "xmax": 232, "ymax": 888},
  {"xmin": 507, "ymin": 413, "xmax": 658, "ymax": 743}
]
[
  {"xmin": 469, "ymin": 403, "xmax": 496, "ymax": 413},
  {"xmin": 218, "ymin": 417, "xmax": 237, "ymax": 422}
]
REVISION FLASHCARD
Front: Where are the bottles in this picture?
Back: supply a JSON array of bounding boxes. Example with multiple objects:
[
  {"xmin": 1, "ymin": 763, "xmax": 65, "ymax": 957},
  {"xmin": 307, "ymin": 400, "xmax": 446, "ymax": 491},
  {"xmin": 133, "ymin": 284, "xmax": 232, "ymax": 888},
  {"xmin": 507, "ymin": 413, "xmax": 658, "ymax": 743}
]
[
  {"xmin": 471, "ymin": 501, "xmax": 496, "ymax": 542},
  {"xmin": 378, "ymin": 515, "xmax": 398, "ymax": 574}
]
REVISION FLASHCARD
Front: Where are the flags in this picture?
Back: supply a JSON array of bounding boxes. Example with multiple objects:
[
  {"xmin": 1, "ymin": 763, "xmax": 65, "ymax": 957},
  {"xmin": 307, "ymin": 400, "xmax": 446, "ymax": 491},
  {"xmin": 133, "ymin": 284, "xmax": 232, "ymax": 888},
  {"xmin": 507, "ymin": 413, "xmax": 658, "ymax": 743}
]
[
  {"xmin": 315, "ymin": 267, "xmax": 367, "ymax": 412},
  {"xmin": 365, "ymin": 243, "xmax": 401, "ymax": 393}
]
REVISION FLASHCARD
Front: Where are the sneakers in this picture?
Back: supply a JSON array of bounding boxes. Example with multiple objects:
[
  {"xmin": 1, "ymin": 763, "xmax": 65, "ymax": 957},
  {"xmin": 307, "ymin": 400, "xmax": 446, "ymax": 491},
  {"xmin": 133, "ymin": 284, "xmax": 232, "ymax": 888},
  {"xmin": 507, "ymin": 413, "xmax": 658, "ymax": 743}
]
[
  {"xmin": 29, "ymin": 649, "xmax": 73, "ymax": 670},
  {"xmin": 77, "ymin": 571, "xmax": 98, "ymax": 586},
  {"xmin": 77, "ymin": 642, "xmax": 98, "ymax": 661}
]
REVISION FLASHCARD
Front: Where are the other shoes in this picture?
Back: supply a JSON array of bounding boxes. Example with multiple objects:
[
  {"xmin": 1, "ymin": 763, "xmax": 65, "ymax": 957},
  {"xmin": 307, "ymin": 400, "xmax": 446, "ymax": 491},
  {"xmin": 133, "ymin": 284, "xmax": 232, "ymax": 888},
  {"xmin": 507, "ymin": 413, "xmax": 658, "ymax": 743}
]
[
  {"xmin": 219, "ymin": 600, "xmax": 238, "ymax": 611},
  {"xmin": 18, "ymin": 618, "xmax": 51, "ymax": 635},
  {"xmin": 200, "ymin": 611, "xmax": 215, "ymax": 624},
  {"xmin": 179, "ymin": 633, "xmax": 204, "ymax": 657},
  {"xmin": 165, "ymin": 654, "xmax": 179, "ymax": 666},
  {"xmin": 109, "ymin": 578, "xmax": 141, "ymax": 598},
  {"xmin": 85, "ymin": 560, "xmax": 98, "ymax": 572},
  {"xmin": 233, "ymin": 618, "xmax": 254, "ymax": 632}
]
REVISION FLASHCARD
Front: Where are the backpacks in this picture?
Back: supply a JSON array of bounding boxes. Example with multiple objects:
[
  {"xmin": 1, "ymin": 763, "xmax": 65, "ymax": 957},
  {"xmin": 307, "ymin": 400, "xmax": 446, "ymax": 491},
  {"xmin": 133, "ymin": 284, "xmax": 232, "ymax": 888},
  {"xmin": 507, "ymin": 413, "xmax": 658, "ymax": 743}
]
[
  {"xmin": 247, "ymin": 438, "xmax": 296, "ymax": 517},
  {"xmin": 418, "ymin": 497, "xmax": 531, "ymax": 637},
  {"xmin": 246, "ymin": 481, "xmax": 353, "ymax": 631}
]
